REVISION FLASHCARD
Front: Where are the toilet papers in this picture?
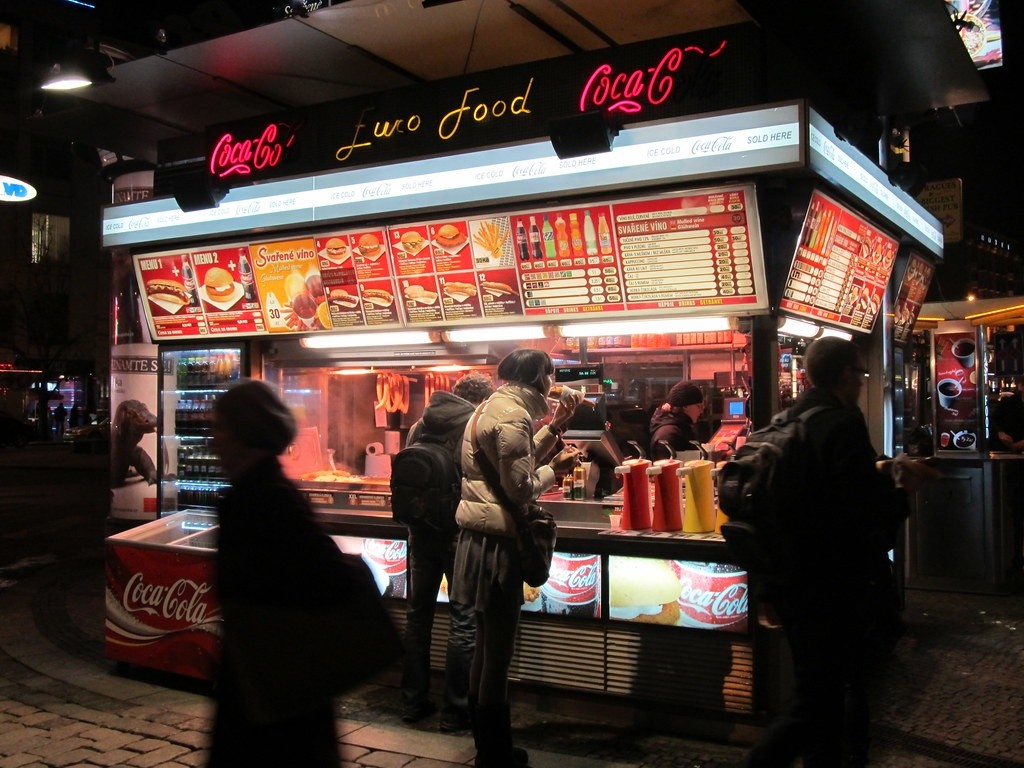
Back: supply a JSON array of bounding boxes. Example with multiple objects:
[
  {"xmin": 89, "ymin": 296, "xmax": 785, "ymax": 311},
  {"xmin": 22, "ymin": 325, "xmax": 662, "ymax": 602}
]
[{"xmin": 365, "ymin": 441, "xmax": 384, "ymax": 456}]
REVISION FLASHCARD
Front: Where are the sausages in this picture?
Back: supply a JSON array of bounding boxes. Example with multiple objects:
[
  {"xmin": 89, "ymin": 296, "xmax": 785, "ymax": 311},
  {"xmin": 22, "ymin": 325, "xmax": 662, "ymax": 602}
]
[
  {"xmin": 375, "ymin": 372, "xmax": 410, "ymax": 414},
  {"xmin": 327, "ymin": 295, "xmax": 357, "ymax": 304},
  {"xmin": 424, "ymin": 372, "xmax": 451, "ymax": 407}
]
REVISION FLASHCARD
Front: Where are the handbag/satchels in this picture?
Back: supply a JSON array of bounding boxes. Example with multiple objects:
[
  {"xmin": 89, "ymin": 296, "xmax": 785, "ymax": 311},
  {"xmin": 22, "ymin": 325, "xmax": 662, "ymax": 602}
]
[
  {"xmin": 252, "ymin": 530, "xmax": 406, "ymax": 713},
  {"xmin": 511, "ymin": 502, "xmax": 559, "ymax": 586}
]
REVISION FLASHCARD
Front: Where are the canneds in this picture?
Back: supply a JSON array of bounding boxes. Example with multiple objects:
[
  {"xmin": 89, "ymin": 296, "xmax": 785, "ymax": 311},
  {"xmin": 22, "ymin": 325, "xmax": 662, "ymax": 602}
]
[
  {"xmin": 180, "ymin": 489, "xmax": 219, "ymax": 507},
  {"xmin": 177, "ymin": 445, "xmax": 225, "ymax": 478},
  {"xmin": 563, "ymin": 477, "xmax": 574, "ymax": 499},
  {"xmin": 573, "ymin": 466, "xmax": 586, "ymax": 500}
]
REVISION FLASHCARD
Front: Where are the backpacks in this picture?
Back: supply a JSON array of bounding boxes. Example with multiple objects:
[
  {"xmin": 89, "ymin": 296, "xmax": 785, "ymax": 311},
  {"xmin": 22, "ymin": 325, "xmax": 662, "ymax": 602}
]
[
  {"xmin": 714, "ymin": 404, "xmax": 839, "ymax": 570},
  {"xmin": 389, "ymin": 418, "xmax": 465, "ymax": 532}
]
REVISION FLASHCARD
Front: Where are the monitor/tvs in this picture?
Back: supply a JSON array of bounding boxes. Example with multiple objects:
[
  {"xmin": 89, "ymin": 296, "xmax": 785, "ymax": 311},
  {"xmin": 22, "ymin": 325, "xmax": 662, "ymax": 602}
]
[{"xmin": 561, "ymin": 428, "xmax": 624, "ymax": 469}]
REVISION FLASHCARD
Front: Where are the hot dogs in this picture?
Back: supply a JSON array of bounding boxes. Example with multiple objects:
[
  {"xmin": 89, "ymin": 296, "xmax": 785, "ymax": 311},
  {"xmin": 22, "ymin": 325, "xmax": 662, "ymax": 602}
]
[
  {"xmin": 362, "ymin": 281, "xmax": 520, "ymax": 305},
  {"xmin": 146, "ymin": 279, "xmax": 191, "ymax": 305}
]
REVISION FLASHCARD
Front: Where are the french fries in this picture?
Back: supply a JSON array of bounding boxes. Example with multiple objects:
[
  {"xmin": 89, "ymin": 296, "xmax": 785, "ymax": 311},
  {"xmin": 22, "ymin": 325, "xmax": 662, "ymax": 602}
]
[{"xmin": 472, "ymin": 220, "xmax": 509, "ymax": 252}]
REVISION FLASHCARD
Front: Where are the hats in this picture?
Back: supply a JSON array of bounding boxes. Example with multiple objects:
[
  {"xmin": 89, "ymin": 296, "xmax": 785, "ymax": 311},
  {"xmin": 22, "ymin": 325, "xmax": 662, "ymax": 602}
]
[{"xmin": 667, "ymin": 381, "xmax": 703, "ymax": 406}]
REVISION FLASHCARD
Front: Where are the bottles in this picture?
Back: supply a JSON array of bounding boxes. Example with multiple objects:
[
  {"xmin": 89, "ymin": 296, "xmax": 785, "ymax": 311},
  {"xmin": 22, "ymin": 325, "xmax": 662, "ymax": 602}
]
[
  {"xmin": 582, "ymin": 210, "xmax": 598, "ymax": 256},
  {"xmin": 516, "ymin": 218, "xmax": 529, "ymax": 261},
  {"xmin": 238, "ymin": 248, "xmax": 255, "ymax": 303},
  {"xmin": 176, "ymin": 355, "xmax": 240, "ymax": 507},
  {"xmin": 528, "ymin": 217, "xmax": 543, "ymax": 259},
  {"xmin": 542, "ymin": 215, "xmax": 556, "ymax": 259},
  {"xmin": 569, "ymin": 213, "xmax": 583, "ymax": 257},
  {"xmin": 597, "ymin": 212, "xmax": 612, "ymax": 255},
  {"xmin": 180, "ymin": 254, "xmax": 198, "ymax": 307},
  {"xmin": 801, "ymin": 199, "xmax": 838, "ymax": 257},
  {"xmin": 555, "ymin": 213, "xmax": 570, "ymax": 258}
]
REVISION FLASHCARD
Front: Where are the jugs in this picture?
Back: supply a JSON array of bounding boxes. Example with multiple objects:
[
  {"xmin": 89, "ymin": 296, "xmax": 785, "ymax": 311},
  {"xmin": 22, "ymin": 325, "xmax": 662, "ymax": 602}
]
[{"xmin": 614, "ymin": 440, "xmax": 738, "ymax": 535}]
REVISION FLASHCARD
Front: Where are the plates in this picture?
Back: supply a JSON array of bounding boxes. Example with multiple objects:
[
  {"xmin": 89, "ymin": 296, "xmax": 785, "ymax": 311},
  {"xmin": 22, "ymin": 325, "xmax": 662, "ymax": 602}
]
[
  {"xmin": 352, "ymin": 245, "xmax": 385, "ymax": 262},
  {"xmin": 317, "ymin": 246, "xmax": 351, "ymax": 265},
  {"xmin": 444, "ymin": 291, "xmax": 470, "ymax": 303},
  {"xmin": 849, "ymin": 241, "xmax": 931, "ymax": 324},
  {"xmin": 147, "ymin": 292, "xmax": 193, "ymax": 314},
  {"xmin": 431, "ymin": 237, "xmax": 469, "ymax": 255},
  {"xmin": 198, "ymin": 281, "xmax": 244, "ymax": 311},
  {"xmin": 392, "ymin": 240, "xmax": 429, "ymax": 256}
]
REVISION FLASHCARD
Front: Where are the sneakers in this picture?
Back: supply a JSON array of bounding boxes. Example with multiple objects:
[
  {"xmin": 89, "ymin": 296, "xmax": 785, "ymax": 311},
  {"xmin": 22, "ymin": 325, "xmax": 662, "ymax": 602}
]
[
  {"xmin": 402, "ymin": 696, "xmax": 436, "ymax": 721},
  {"xmin": 439, "ymin": 708, "xmax": 470, "ymax": 733}
]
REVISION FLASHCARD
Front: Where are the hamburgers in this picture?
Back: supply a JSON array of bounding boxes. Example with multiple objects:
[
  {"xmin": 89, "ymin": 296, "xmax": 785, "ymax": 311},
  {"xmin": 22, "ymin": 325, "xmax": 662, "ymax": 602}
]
[
  {"xmin": 204, "ymin": 267, "xmax": 235, "ymax": 302},
  {"xmin": 850, "ymin": 238, "xmax": 892, "ymax": 312},
  {"xmin": 608, "ymin": 556, "xmax": 684, "ymax": 626},
  {"xmin": 326, "ymin": 225, "xmax": 467, "ymax": 260}
]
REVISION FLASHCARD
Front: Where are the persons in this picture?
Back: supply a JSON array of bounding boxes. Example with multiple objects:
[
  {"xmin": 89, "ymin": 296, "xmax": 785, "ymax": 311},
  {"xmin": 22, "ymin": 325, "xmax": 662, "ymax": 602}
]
[
  {"xmin": 990, "ymin": 376, "xmax": 1024, "ymax": 452},
  {"xmin": 202, "ymin": 380, "xmax": 348, "ymax": 768},
  {"xmin": 54, "ymin": 403, "xmax": 67, "ymax": 435},
  {"xmin": 444, "ymin": 349, "xmax": 580, "ymax": 768},
  {"xmin": 83, "ymin": 404, "xmax": 97, "ymax": 424},
  {"xmin": 650, "ymin": 381, "xmax": 704, "ymax": 460},
  {"xmin": 400, "ymin": 371, "xmax": 495, "ymax": 730},
  {"xmin": 745, "ymin": 338, "xmax": 915, "ymax": 768},
  {"xmin": 69, "ymin": 404, "xmax": 79, "ymax": 427}
]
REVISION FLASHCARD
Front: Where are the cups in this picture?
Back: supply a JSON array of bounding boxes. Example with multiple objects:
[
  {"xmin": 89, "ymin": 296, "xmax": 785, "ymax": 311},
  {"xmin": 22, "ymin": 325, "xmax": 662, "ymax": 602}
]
[
  {"xmin": 953, "ymin": 430, "xmax": 976, "ymax": 450},
  {"xmin": 937, "ymin": 378, "xmax": 962, "ymax": 408},
  {"xmin": 951, "ymin": 338, "xmax": 976, "ymax": 368},
  {"xmin": 284, "ymin": 272, "xmax": 325, "ymax": 327}
]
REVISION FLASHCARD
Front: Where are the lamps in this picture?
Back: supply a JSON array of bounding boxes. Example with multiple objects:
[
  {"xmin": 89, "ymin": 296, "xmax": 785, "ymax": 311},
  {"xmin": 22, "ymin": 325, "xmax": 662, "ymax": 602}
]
[
  {"xmin": 557, "ymin": 315, "xmax": 739, "ymax": 339},
  {"xmin": 298, "ymin": 328, "xmax": 440, "ymax": 348},
  {"xmin": 42, "ymin": 42, "xmax": 117, "ymax": 94},
  {"xmin": 829, "ymin": 102, "xmax": 884, "ymax": 153},
  {"xmin": 442, "ymin": 324, "xmax": 555, "ymax": 342},
  {"xmin": 174, "ymin": 170, "xmax": 232, "ymax": 213},
  {"xmin": 547, "ymin": 100, "xmax": 622, "ymax": 159}
]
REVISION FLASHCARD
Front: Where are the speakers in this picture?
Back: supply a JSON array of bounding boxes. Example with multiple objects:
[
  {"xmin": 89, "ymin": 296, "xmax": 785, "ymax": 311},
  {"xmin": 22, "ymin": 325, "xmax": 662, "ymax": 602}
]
[
  {"xmin": 549, "ymin": 109, "xmax": 616, "ymax": 160},
  {"xmin": 170, "ymin": 169, "xmax": 229, "ymax": 213}
]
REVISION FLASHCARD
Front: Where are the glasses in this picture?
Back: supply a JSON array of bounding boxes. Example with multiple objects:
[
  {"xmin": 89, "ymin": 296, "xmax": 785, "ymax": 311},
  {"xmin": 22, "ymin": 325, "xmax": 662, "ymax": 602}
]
[{"xmin": 844, "ymin": 365, "xmax": 872, "ymax": 379}]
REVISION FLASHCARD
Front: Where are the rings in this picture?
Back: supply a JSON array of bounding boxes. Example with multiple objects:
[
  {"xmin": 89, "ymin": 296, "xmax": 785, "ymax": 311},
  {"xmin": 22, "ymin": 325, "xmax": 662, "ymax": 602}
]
[{"xmin": 569, "ymin": 404, "xmax": 572, "ymax": 407}]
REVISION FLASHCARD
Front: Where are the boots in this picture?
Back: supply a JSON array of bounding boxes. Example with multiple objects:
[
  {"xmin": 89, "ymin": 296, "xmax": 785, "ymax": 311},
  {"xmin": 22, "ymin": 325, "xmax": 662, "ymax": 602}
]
[{"xmin": 469, "ymin": 701, "xmax": 528, "ymax": 768}]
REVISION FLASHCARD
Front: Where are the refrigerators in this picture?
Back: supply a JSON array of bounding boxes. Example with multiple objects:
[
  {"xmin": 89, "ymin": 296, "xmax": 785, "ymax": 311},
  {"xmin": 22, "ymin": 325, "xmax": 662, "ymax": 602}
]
[
  {"xmin": 103, "ymin": 509, "xmax": 221, "ymax": 682},
  {"xmin": 156, "ymin": 345, "xmax": 248, "ymax": 518}
]
